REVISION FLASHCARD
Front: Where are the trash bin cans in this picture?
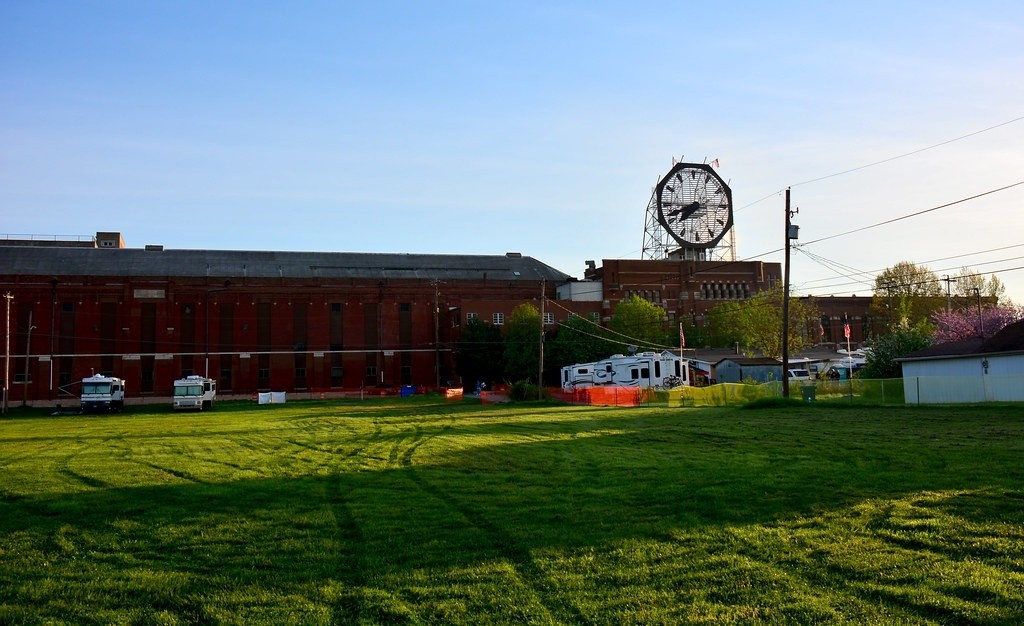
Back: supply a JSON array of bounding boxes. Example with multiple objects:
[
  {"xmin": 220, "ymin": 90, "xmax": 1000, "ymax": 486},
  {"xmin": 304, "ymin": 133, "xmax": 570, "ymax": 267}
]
[
  {"xmin": 800, "ymin": 386, "xmax": 817, "ymax": 401},
  {"xmin": 401, "ymin": 386, "xmax": 416, "ymax": 398}
]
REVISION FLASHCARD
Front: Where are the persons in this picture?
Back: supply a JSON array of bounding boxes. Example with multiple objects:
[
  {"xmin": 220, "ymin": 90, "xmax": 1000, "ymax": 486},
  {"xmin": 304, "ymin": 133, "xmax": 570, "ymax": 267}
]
[{"xmin": 476, "ymin": 380, "xmax": 481, "ymax": 395}]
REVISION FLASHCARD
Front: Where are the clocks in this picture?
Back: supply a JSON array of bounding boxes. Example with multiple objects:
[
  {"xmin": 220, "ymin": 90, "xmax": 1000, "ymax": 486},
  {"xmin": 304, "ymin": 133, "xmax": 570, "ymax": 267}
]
[{"xmin": 657, "ymin": 163, "xmax": 733, "ymax": 250}]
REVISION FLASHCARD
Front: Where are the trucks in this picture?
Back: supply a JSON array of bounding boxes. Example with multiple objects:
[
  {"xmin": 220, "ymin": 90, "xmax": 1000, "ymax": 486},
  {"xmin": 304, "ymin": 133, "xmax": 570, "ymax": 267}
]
[
  {"xmin": 173, "ymin": 375, "xmax": 218, "ymax": 409},
  {"xmin": 81, "ymin": 375, "xmax": 125, "ymax": 415}
]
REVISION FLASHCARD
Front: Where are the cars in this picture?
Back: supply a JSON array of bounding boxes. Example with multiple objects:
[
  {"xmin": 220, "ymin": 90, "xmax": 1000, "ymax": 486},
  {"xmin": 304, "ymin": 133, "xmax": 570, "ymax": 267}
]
[{"xmin": 787, "ymin": 369, "xmax": 812, "ymax": 382}]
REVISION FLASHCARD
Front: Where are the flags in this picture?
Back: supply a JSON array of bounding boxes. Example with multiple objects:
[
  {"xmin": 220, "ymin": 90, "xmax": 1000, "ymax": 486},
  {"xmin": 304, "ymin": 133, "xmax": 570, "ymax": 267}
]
[
  {"xmin": 819, "ymin": 324, "xmax": 824, "ymax": 336},
  {"xmin": 844, "ymin": 315, "xmax": 851, "ymax": 337},
  {"xmin": 680, "ymin": 328, "xmax": 684, "ymax": 346}
]
